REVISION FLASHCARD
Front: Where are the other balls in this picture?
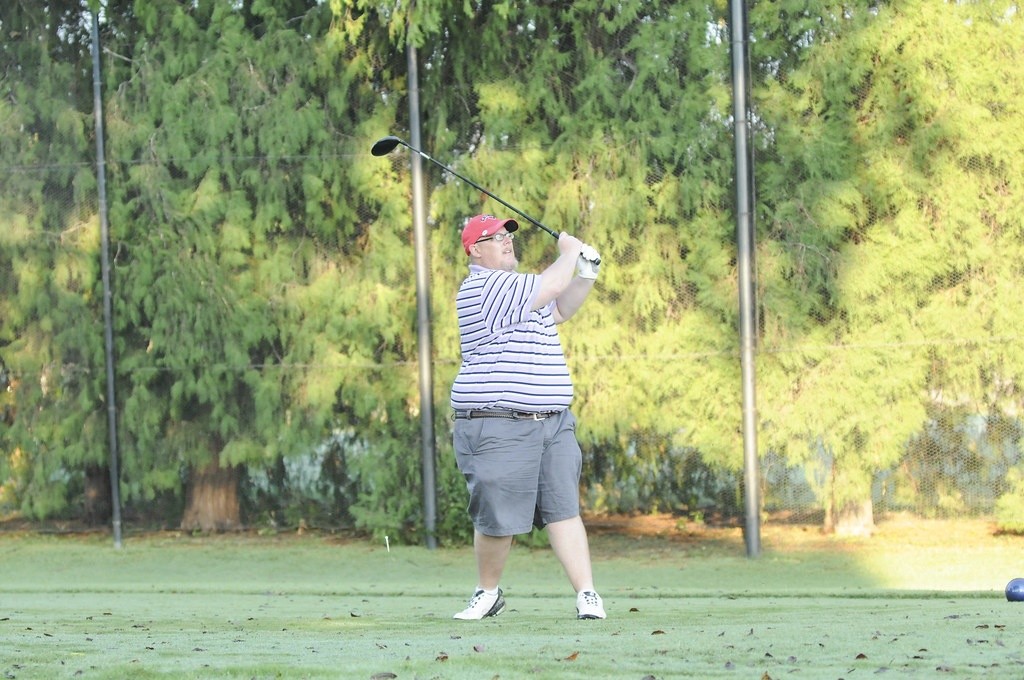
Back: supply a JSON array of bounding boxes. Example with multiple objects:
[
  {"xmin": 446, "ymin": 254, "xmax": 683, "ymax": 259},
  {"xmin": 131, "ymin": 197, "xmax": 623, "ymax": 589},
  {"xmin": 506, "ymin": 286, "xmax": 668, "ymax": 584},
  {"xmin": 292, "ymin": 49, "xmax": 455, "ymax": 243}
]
[{"xmin": 1005, "ymin": 578, "xmax": 1024, "ymax": 602}]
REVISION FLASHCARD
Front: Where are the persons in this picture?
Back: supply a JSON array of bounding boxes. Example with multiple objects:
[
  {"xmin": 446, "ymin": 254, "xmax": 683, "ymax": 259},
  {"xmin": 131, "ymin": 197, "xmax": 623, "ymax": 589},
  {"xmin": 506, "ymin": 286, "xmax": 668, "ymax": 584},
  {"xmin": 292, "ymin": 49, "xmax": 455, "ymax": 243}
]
[{"xmin": 448, "ymin": 214, "xmax": 606, "ymax": 619}]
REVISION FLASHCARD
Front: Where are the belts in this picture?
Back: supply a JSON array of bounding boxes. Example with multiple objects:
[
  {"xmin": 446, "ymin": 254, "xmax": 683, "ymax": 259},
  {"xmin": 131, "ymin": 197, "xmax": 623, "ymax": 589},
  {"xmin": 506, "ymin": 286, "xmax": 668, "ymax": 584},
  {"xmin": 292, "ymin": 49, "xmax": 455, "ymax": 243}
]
[{"xmin": 452, "ymin": 409, "xmax": 560, "ymax": 423}]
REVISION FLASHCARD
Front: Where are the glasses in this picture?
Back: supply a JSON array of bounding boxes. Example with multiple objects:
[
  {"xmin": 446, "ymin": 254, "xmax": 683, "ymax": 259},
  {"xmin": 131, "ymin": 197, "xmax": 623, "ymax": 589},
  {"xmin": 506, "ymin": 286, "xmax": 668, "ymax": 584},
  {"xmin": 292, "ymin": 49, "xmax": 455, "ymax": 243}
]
[{"xmin": 475, "ymin": 231, "xmax": 515, "ymax": 246}]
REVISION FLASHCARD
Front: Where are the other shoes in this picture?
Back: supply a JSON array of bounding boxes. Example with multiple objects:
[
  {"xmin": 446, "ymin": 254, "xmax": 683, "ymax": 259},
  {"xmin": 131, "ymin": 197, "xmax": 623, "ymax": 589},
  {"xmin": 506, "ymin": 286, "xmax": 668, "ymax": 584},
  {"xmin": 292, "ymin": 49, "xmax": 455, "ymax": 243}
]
[
  {"xmin": 573, "ymin": 590, "xmax": 606, "ymax": 620},
  {"xmin": 455, "ymin": 586, "xmax": 507, "ymax": 621}
]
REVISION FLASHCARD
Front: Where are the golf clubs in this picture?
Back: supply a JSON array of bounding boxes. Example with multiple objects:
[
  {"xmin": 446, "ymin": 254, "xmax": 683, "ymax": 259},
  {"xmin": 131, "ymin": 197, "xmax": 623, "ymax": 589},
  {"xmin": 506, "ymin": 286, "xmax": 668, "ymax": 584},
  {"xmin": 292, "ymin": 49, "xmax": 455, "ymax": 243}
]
[{"xmin": 370, "ymin": 136, "xmax": 601, "ymax": 265}]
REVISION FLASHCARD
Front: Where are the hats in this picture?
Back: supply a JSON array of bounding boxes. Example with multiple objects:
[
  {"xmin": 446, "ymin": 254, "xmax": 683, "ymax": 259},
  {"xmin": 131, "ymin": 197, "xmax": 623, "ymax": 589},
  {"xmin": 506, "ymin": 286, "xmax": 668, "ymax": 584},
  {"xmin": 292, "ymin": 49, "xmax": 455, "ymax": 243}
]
[{"xmin": 460, "ymin": 215, "xmax": 520, "ymax": 254}]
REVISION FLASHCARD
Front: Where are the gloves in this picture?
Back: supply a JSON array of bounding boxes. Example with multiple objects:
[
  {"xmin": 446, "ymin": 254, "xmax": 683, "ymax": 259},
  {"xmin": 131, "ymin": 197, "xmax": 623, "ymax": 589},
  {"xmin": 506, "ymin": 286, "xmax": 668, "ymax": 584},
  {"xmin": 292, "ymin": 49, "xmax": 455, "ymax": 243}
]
[{"xmin": 576, "ymin": 243, "xmax": 602, "ymax": 279}]
